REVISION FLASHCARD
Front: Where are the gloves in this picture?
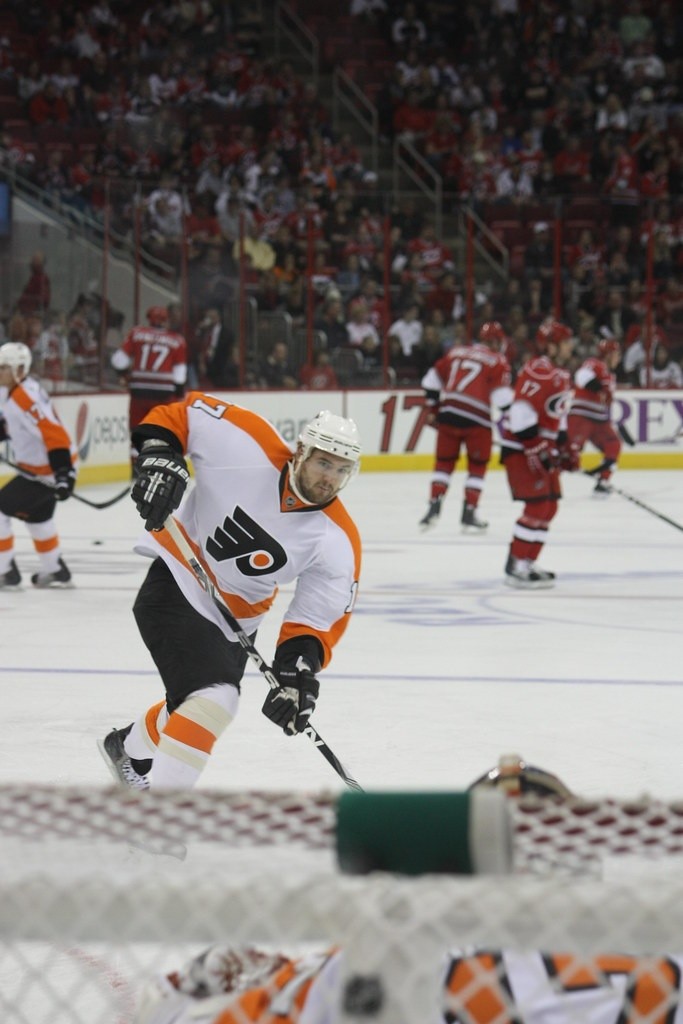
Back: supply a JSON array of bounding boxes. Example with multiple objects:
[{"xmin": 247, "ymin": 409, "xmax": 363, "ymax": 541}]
[
  {"xmin": 132, "ymin": 445, "xmax": 189, "ymax": 532},
  {"xmin": 525, "ymin": 439, "xmax": 561, "ymax": 475},
  {"xmin": 54, "ymin": 467, "xmax": 75, "ymax": 500},
  {"xmin": 262, "ymin": 663, "xmax": 320, "ymax": 736}
]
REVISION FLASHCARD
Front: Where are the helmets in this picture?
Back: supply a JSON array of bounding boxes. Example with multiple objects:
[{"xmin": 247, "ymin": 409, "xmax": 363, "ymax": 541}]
[
  {"xmin": 147, "ymin": 307, "xmax": 168, "ymax": 327},
  {"xmin": 598, "ymin": 339, "xmax": 622, "ymax": 356},
  {"xmin": 467, "ymin": 765, "xmax": 575, "ymax": 803},
  {"xmin": 298, "ymin": 410, "xmax": 362, "ymax": 462},
  {"xmin": 481, "ymin": 322, "xmax": 503, "ymax": 340},
  {"xmin": 0, "ymin": 342, "xmax": 32, "ymax": 375},
  {"xmin": 536, "ymin": 320, "xmax": 573, "ymax": 351}
]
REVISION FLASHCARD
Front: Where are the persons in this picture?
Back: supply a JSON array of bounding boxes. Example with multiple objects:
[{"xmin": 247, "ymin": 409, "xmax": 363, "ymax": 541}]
[
  {"xmin": 420, "ymin": 321, "xmax": 511, "ymax": 535},
  {"xmin": 165, "ymin": 755, "xmax": 678, "ymax": 1024},
  {"xmin": 96, "ymin": 394, "xmax": 361, "ymax": 792},
  {"xmin": 0, "ymin": 343, "xmax": 77, "ymax": 591},
  {"xmin": 499, "ymin": 322, "xmax": 579, "ymax": 589},
  {"xmin": 111, "ymin": 306, "xmax": 188, "ymax": 486},
  {"xmin": 565, "ymin": 340, "xmax": 621, "ymax": 493},
  {"xmin": 0, "ymin": 0, "xmax": 683, "ymax": 389}
]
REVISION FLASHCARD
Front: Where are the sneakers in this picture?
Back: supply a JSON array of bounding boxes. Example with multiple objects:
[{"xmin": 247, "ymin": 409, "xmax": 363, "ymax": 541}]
[
  {"xmin": 505, "ymin": 554, "xmax": 556, "ymax": 588},
  {"xmin": 31, "ymin": 558, "xmax": 72, "ymax": 589},
  {"xmin": 420, "ymin": 498, "xmax": 443, "ymax": 525},
  {"xmin": 595, "ymin": 480, "xmax": 614, "ymax": 494},
  {"xmin": 462, "ymin": 502, "xmax": 488, "ymax": 528},
  {"xmin": 96, "ymin": 721, "xmax": 153, "ymax": 792},
  {"xmin": 0, "ymin": 559, "xmax": 21, "ymax": 589}
]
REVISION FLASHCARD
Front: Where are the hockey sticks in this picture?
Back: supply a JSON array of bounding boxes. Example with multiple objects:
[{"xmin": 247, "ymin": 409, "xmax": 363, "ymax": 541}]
[
  {"xmin": 0, "ymin": 453, "xmax": 133, "ymax": 511},
  {"xmin": 575, "ymin": 462, "xmax": 683, "ymax": 531},
  {"xmin": 165, "ymin": 510, "xmax": 366, "ymax": 796}
]
